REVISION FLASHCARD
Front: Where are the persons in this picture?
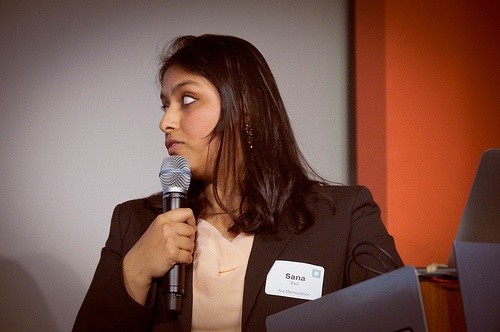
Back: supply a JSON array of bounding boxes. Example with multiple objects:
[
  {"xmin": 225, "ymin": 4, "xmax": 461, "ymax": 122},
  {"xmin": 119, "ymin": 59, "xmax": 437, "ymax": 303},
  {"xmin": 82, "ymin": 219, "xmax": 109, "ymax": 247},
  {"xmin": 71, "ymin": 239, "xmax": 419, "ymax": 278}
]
[{"xmin": 70, "ymin": 34, "xmax": 414, "ymax": 332}]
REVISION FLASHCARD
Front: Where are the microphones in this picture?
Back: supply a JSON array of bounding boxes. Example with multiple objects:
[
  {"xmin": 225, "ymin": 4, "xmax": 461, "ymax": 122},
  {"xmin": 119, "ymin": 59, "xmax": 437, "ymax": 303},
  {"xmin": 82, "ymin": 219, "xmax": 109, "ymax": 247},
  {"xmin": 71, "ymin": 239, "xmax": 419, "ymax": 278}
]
[{"xmin": 159, "ymin": 156, "xmax": 192, "ymax": 314}]
